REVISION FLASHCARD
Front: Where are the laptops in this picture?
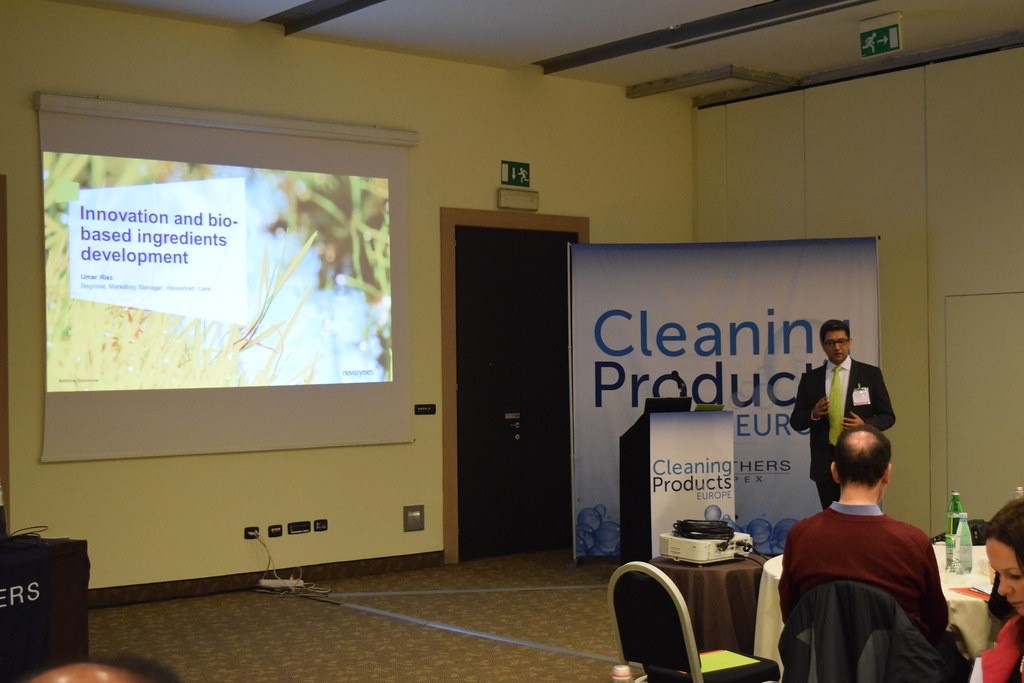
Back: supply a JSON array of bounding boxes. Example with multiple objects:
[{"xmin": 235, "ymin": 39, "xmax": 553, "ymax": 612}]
[{"xmin": 644, "ymin": 397, "xmax": 693, "ymax": 413}]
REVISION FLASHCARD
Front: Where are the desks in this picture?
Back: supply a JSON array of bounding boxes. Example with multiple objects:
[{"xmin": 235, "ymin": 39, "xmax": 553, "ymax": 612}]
[
  {"xmin": 650, "ymin": 554, "xmax": 772, "ymax": 656},
  {"xmin": 754, "ymin": 542, "xmax": 995, "ymax": 683}
]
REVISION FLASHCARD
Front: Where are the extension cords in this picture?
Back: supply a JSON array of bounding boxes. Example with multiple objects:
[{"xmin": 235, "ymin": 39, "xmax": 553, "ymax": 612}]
[{"xmin": 259, "ymin": 579, "xmax": 305, "ymax": 588}]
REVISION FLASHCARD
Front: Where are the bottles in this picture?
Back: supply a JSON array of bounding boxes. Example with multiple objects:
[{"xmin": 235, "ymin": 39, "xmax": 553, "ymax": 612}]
[
  {"xmin": 611, "ymin": 664, "xmax": 634, "ymax": 683},
  {"xmin": 946, "ymin": 490, "xmax": 964, "ymax": 563},
  {"xmin": 954, "ymin": 513, "xmax": 972, "ymax": 575},
  {"xmin": 1014, "ymin": 486, "xmax": 1024, "ymax": 499}
]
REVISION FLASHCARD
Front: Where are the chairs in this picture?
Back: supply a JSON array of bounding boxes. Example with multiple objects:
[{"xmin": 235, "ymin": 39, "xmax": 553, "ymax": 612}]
[
  {"xmin": 777, "ymin": 582, "xmax": 972, "ymax": 683},
  {"xmin": 607, "ymin": 562, "xmax": 781, "ymax": 683}
]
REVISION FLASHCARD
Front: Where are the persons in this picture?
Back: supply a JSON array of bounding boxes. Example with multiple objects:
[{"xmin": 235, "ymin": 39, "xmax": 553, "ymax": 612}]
[
  {"xmin": 778, "ymin": 424, "xmax": 951, "ymax": 648},
  {"xmin": 789, "ymin": 320, "xmax": 898, "ymax": 510},
  {"xmin": 965, "ymin": 497, "xmax": 1024, "ymax": 683},
  {"xmin": 19, "ymin": 653, "xmax": 184, "ymax": 682}
]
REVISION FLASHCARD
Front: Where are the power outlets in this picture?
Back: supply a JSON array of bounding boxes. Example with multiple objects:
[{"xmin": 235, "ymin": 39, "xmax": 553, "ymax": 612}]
[
  {"xmin": 244, "ymin": 527, "xmax": 260, "ymax": 539},
  {"xmin": 288, "ymin": 521, "xmax": 311, "ymax": 534},
  {"xmin": 268, "ymin": 525, "xmax": 283, "ymax": 537},
  {"xmin": 314, "ymin": 519, "xmax": 328, "ymax": 531}
]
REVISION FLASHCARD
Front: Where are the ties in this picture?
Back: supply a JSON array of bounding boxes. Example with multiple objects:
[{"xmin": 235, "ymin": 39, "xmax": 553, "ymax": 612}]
[{"xmin": 828, "ymin": 366, "xmax": 844, "ymax": 446}]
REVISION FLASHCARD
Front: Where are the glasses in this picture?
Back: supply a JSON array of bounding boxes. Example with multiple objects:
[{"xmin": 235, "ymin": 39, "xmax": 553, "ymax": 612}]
[{"xmin": 825, "ymin": 339, "xmax": 849, "ymax": 346}]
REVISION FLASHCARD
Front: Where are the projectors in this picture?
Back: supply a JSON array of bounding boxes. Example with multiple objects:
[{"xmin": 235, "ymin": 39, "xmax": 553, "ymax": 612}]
[{"xmin": 658, "ymin": 531, "xmax": 752, "ymax": 565}]
